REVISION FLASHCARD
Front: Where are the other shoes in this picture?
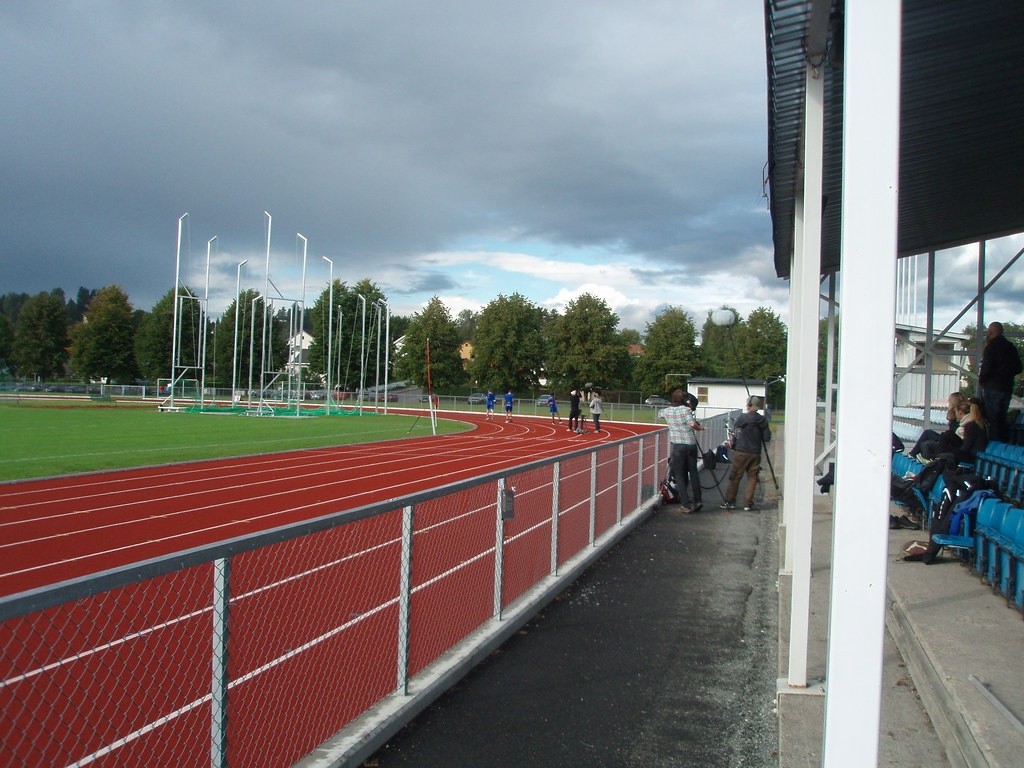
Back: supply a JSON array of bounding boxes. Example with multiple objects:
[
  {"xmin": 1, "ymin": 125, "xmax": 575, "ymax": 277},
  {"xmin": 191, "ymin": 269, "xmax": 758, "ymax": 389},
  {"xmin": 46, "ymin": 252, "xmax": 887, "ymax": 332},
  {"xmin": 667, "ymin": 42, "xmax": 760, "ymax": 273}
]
[
  {"xmin": 744, "ymin": 505, "xmax": 751, "ymax": 511},
  {"xmin": 573, "ymin": 429, "xmax": 577, "ymax": 433},
  {"xmin": 566, "ymin": 429, "xmax": 571, "ymax": 432},
  {"xmin": 509, "ymin": 419, "xmax": 512, "ymax": 423},
  {"xmin": 690, "ymin": 502, "xmax": 703, "ymax": 512},
  {"xmin": 678, "ymin": 505, "xmax": 692, "ymax": 513},
  {"xmin": 900, "ymin": 515, "xmax": 920, "ymax": 529},
  {"xmin": 594, "ymin": 430, "xmax": 599, "ymax": 433},
  {"xmin": 486, "ymin": 418, "xmax": 489, "ymax": 421},
  {"xmin": 720, "ymin": 502, "xmax": 737, "ymax": 509},
  {"xmin": 901, "ymin": 471, "xmax": 916, "ymax": 481},
  {"xmin": 889, "ymin": 514, "xmax": 900, "ymax": 529},
  {"xmin": 552, "ymin": 422, "xmax": 554, "ymax": 425},
  {"xmin": 504, "ymin": 420, "xmax": 508, "ymax": 423},
  {"xmin": 491, "ymin": 417, "xmax": 494, "ymax": 421},
  {"xmin": 558, "ymin": 422, "xmax": 563, "ymax": 424}
]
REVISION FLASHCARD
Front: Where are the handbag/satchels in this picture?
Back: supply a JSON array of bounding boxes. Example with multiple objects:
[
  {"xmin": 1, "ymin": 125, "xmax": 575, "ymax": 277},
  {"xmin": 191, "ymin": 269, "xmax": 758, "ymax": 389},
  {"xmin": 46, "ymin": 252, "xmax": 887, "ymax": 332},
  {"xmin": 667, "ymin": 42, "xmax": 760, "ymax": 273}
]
[{"xmin": 901, "ymin": 540, "xmax": 944, "ymax": 562}]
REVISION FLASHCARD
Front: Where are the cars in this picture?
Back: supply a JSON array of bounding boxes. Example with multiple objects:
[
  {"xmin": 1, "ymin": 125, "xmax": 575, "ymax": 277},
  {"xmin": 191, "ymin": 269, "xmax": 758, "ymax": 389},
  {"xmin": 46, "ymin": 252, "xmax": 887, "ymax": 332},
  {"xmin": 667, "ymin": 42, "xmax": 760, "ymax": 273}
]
[
  {"xmin": 537, "ymin": 395, "xmax": 553, "ymax": 406},
  {"xmin": 468, "ymin": 393, "xmax": 486, "ymax": 404},
  {"xmin": 645, "ymin": 394, "xmax": 661, "ymax": 404},
  {"xmin": 307, "ymin": 391, "xmax": 321, "ymax": 400}
]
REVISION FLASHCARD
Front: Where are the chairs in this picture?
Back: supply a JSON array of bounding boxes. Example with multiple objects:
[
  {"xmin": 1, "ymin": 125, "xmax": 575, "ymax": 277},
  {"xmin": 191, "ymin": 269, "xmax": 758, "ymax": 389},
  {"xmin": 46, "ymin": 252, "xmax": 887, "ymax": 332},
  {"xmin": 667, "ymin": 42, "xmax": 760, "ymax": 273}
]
[{"xmin": 892, "ymin": 422, "xmax": 1024, "ymax": 621}]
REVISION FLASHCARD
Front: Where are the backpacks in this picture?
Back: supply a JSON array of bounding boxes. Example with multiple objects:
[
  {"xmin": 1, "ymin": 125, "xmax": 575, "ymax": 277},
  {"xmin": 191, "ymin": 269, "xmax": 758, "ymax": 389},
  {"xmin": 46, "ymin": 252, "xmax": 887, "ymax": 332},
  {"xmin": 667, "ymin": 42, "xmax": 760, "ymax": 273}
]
[
  {"xmin": 703, "ymin": 449, "xmax": 716, "ymax": 469},
  {"xmin": 659, "ymin": 481, "xmax": 678, "ymax": 505},
  {"xmin": 716, "ymin": 444, "xmax": 729, "ymax": 462}
]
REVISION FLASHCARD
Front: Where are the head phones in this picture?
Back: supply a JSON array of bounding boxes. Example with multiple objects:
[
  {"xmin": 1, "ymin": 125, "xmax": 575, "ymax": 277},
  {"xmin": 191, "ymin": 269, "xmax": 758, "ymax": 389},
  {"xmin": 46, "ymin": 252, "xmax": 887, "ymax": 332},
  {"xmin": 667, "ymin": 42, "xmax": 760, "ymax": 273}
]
[{"xmin": 748, "ymin": 396, "xmax": 754, "ymax": 407}]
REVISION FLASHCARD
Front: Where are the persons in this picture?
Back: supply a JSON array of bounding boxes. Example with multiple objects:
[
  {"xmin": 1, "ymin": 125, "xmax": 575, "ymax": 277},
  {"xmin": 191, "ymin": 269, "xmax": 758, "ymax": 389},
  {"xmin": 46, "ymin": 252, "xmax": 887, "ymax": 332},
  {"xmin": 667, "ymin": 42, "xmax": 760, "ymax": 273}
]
[
  {"xmin": 502, "ymin": 390, "xmax": 514, "ymax": 423},
  {"xmin": 159, "ymin": 383, "xmax": 173, "ymax": 397},
  {"xmin": 589, "ymin": 391, "xmax": 603, "ymax": 433},
  {"xmin": 486, "ymin": 388, "xmax": 497, "ymax": 421},
  {"xmin": 548, "ymin": 393, "xmax": 563, "ymax": 425},
  {"xmin": 720, "ymin": 396, "xmax": 771, "ymax": 511},
  {"xmin": 658, "ymin": 389, "xmax": 705, "ymax": 512},
  {"xmin": 566, "ymin": 390, "xmax": 582, "ymax": 433},
  {"xmin": 892, "ymin": 322, "xmax": 1022, "ymax": 529}
]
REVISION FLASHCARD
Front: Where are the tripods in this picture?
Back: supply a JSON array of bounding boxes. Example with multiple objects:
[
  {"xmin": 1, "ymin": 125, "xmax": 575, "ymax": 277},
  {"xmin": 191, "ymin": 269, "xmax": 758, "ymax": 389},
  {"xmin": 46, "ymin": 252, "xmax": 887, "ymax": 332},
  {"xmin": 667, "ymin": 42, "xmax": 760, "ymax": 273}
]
[{"xmin": 653, "ymin": 420, "xmax": 731, "ymax": 515}]
